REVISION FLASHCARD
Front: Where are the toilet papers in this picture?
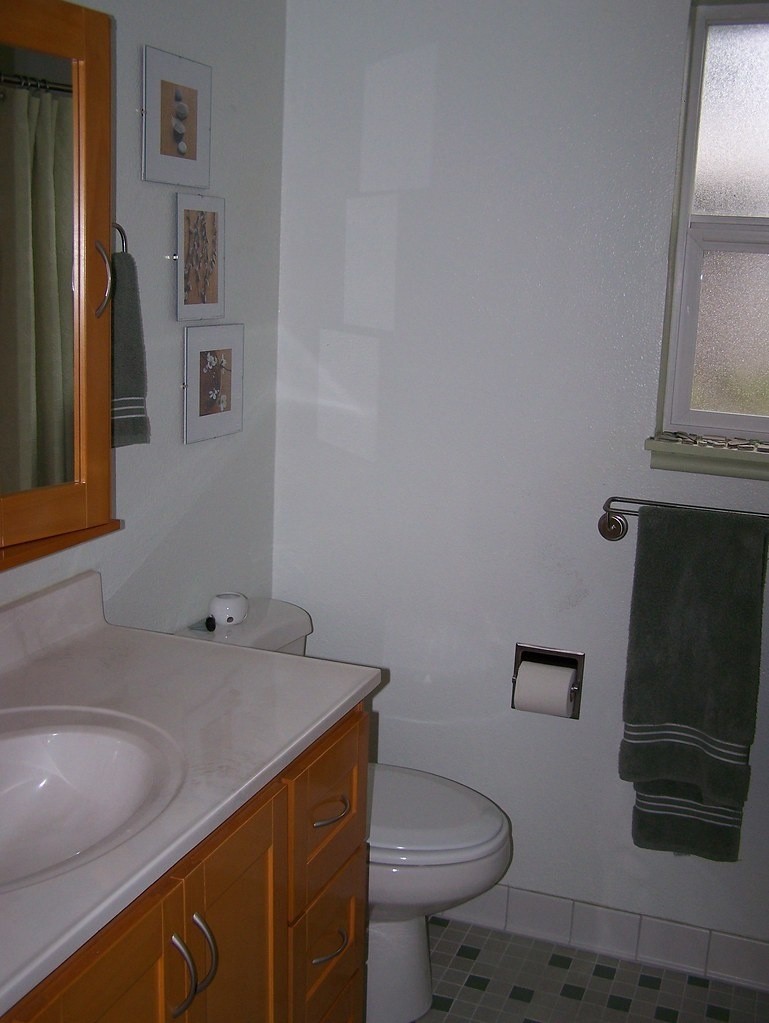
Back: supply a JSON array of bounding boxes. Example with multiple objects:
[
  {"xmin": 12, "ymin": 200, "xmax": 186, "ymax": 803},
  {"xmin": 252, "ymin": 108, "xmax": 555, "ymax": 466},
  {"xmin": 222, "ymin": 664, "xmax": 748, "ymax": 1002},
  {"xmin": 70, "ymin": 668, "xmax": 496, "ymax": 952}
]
[{"xmin": 514, "ymin": 660, "xmax": 577, "ymax": 718}]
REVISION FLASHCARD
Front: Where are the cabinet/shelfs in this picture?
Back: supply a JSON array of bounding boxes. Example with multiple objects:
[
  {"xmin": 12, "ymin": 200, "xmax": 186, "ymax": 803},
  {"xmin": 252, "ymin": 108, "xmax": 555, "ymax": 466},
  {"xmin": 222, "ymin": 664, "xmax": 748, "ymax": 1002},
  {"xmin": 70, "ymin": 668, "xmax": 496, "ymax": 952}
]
[
  {"xmin": 0, "ymin": 699, "xmax": 370, "ymax": 1023},
  {"xmin": 0, "ymin": 0, "xmax": 119, "ymax": 573}
]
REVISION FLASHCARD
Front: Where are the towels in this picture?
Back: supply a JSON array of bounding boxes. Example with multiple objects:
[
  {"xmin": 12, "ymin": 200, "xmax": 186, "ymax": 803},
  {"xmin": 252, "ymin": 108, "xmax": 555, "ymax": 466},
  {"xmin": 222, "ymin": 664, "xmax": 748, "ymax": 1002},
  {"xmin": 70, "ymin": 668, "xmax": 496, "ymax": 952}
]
[
  {"xmin": 110, "ymin": 250, "xmax": 151, "ymax": 449},
  {"xmin": 617, "ymin": 505, "xmax": 769, "ymax": 864}
]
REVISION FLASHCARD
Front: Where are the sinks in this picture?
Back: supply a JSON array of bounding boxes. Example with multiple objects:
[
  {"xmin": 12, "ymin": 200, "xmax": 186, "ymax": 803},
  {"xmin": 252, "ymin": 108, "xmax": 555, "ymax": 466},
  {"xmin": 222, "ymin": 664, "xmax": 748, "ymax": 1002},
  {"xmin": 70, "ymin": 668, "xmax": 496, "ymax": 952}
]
[{"xmin": 0, "ymin": 704, "xmax": 189, "ymax": 894}]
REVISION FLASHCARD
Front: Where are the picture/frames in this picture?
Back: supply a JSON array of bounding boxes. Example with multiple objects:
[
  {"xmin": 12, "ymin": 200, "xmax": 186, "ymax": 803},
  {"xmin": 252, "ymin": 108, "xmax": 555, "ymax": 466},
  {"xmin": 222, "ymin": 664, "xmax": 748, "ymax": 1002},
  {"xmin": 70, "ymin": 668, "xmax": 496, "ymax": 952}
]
[
  {"xmin": 141, "ymin": 44, "xmax": 213, "ymax": 189},
  {"xmin": 177, "ymin": 193, "xmax": 226, "ymax": 322},
  {"xmin": 183, "ymin": 323, "xmax": 245, "ymax": 445}
]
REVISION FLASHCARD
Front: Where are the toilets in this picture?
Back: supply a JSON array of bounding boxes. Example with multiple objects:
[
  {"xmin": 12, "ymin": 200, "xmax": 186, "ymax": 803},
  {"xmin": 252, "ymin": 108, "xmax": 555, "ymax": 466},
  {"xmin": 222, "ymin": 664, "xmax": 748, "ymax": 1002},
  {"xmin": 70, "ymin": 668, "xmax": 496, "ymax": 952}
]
[{"xmin": 171, "ymin": 594, "xmax": 515, "ymax": 1023}]
